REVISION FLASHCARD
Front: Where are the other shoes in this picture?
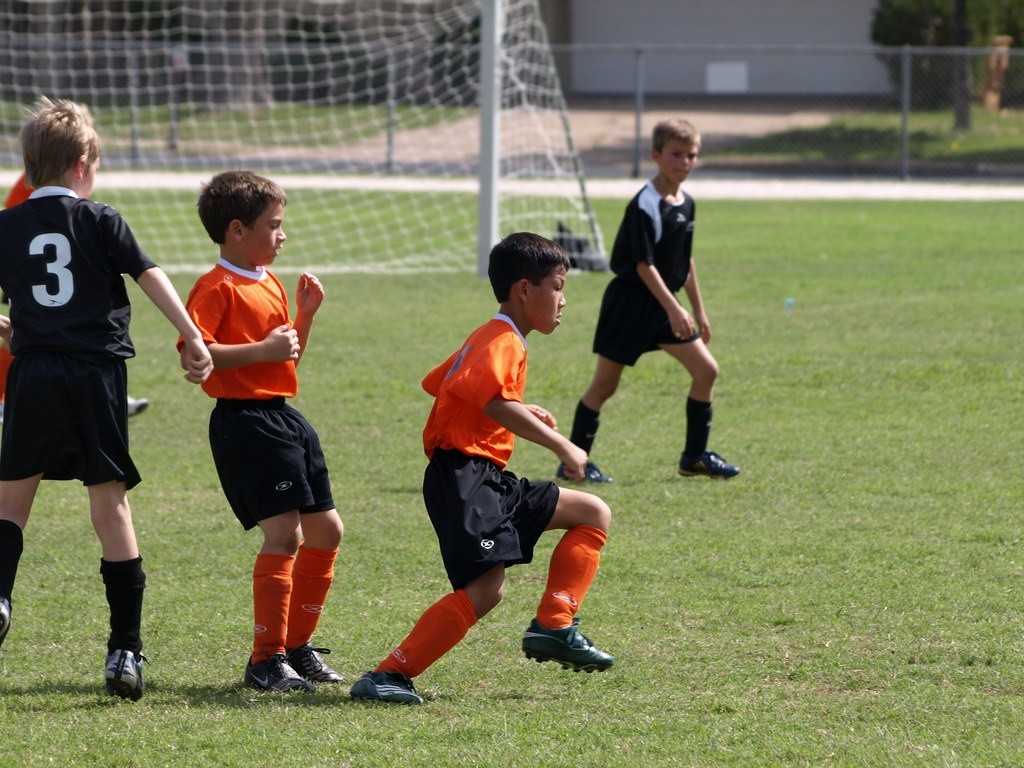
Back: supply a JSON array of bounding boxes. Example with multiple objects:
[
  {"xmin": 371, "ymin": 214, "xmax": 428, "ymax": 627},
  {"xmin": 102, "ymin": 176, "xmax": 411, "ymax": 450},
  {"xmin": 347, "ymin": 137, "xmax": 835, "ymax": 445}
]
[{"xmin": 127, "ymin": 393, "xmax": 150, "ymax": 417}]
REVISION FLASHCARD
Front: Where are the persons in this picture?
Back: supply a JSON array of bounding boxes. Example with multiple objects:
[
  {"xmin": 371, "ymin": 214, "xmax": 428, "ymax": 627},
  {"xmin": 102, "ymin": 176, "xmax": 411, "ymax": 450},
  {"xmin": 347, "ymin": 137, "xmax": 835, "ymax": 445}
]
[
  {"xmin": 557, "ymin": 116, "xmax": 740, "ymax": 484},
  {"xmin": 0, "ymin": 109, "xmax": 215, "ymax": 700},
  {"xmin": 0, "ymin": 97, "xmax": 148, "ymax": 420},
  {"xmin": 177, "ymin": 168, "xmax": 342, "ymax": 693},
  {"xmin": 348, "ymin": 232, "xmax": 613, "ymax": 706}
]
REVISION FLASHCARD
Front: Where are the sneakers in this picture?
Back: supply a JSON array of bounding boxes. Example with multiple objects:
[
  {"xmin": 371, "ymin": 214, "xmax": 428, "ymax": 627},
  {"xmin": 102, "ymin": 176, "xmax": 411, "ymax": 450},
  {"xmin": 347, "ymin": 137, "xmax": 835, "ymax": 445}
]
[
  {"xmin": 522, "ymin": 617, "xmax": 615, "ymax": 674},
  {"xmin": 556, "ymin": 460, "xmax": 615, "ymax": 485},
  {"xmin": 0, "ymin": 595, "xmax": 12, "ymax": 648},
  {"xmin": 285, "ymin": 642, "xmax": 350, "ymax": 688},
  {"xmin": 245, "ymin": 653, "xmax": 316, "ymax": 694},
  {"xmin": 678, "ymin": 451, "xmax": 740, "ymax": 480},
  {"xmin": 104, "ymin": 648, "xmax": 151, "ymax": 703},
  {"xmin": 350, "ymin": 670, "xmax": 425, "ymax": 706}
]
